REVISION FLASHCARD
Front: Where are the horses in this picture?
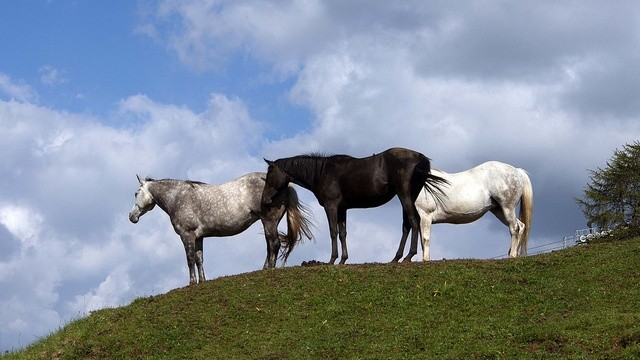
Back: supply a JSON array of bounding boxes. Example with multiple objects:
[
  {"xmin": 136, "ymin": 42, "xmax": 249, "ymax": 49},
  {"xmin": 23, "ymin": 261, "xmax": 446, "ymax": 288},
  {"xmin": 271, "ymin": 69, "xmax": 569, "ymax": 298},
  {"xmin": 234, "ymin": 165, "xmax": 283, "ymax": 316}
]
[
  {"xmin": 414, "ymin": 160, "xmax": 534, "ymax": 262},
  {"xmin": 129, "ymin": 174, "xmax": 318, "ymax": 285},
  {"xmin": 261, "ymin": 147, "xmax": 430, "ymax": 270}
]
